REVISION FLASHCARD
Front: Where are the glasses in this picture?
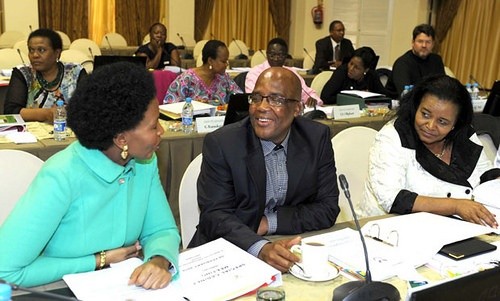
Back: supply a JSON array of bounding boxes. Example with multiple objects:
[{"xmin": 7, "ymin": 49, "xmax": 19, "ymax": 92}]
[
  {"xmin": 247, "ymin": 93, "xmax": 300, "ymax": 107},
  {"xmin": 364, "ymin": 224, "xmax": 399, "ymax": 247},
  {"xmin": 266, "ymin": 52, "xmax": 286, "ymax": 58}
]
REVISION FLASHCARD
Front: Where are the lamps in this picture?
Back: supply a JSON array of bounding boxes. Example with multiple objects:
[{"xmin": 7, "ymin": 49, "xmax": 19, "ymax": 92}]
[{"xmin": 311, "ymin": 5, "xmax": 323, "ymax": 24}]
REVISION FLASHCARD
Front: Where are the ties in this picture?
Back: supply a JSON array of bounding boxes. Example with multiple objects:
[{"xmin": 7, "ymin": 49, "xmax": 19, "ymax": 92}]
[{"xmin": 334, "ymin": 45, "xmax": 341, "ymax": 62}]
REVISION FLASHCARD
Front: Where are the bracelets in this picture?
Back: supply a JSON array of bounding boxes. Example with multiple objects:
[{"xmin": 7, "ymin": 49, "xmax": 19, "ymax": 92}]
[{"xmin": 99, "ymin": 251, "xmax": 106, "ymax": 269}]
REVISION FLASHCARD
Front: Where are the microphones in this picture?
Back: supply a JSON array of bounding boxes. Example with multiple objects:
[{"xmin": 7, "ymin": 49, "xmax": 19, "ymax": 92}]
[
  {"xmin": 18, "ymin": 49, "xmax": 27, "ymax": 67},
  {"xmin": 303, "ymin": 49, "xmax": 315, "ymax": 64},
  {"xmin": 106, "ymin": 36, "xmax": 114, "ymax": 54},
  {"xmin": 231, "ymin": 38, "xmax": 247, "ymax": 59},
  {"xmin": 177, "ymin": 33, "xmax": 192, "ymax": 59},
  {"xmin": 29, "ymin": 25, "xmax": 33, "ymax": 34},
  {"xmin": 302, "ymin": 88, "xmax": 326, "ymax": 120},
  {"xmin": 331, "ymin": 173, "xmax": 400, "ymax": 301},
  {"xmin": 89, "ymin": 48, "xmax": 94, "ymax": 60}
]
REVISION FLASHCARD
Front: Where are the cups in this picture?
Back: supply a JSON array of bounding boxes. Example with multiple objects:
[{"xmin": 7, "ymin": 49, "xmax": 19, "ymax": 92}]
[
  {"xmin": 256, "ymin": 287, "xmax": 285, "ymax": 301},
  {"xmin": 291, "ymin": 238, "xmax": 329, "ymax": 279}
]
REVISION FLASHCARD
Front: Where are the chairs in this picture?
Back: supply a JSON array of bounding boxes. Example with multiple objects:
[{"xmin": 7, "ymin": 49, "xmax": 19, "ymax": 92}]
[
  {"xmin": 332, "ymin": 126, "xmax": 388, "ymax": 223},
  {"xmin": 0, "ymin": 150, "xmax": 44, "ymax": 228},
  {"xmin": 194, "ymin": 40, "xmax": 266, "ymax": 69},
  {"xmin": 0, "ymin": 31, "xmax": 127, "ymax": 75}
]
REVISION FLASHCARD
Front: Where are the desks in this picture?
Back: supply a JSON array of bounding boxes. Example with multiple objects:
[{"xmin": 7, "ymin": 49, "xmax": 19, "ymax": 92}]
[
  {"xmin": 0, "ymin": 118, "xmax": 397, "ymax": 227},
  {"xmin": 0, "ymin": 212, "xmax": 500, "ymax": 301}
]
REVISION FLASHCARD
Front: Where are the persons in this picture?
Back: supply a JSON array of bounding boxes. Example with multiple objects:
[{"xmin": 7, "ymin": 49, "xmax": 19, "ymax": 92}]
[
  {"xmin": 355, "ymin": 75, "xmax": 500, "ymax": 228},
  {"xmin": 188, "ymin": 66, "xmax": 341, "ymax": 274},
  {"xmin": 386, "ymin": 24, "xmax": 446, "ymax": 99},
  {"xmin": 320, "ymin": 47, "xmax": 386, "ymax": 105},
  {"xmin": 312, "ymin": 20, "xmax": 354, "ymax": 75},
  {"xmin": 4, "ymin": 29, "xmax": 88, "ymax": 122},
  {"xmin": 0, "ymin": 61, "xmax": 181, "ymax": 291},
  {"xmin": 245, "ymin": 38, "xmax": 323, "ymax": 108},
  {"xmin": 136, "ymin": 22, "xmax": 181, "ymax": 69},
  {"xmin": 163, "ymin": 40, "xmax": 244, "ymax": 104}
]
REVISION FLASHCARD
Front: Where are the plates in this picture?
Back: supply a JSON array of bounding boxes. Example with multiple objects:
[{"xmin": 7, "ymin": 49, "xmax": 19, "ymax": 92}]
[{"xmin": 289, "ymin": 260, "xmax": 341, "ymax": 282}]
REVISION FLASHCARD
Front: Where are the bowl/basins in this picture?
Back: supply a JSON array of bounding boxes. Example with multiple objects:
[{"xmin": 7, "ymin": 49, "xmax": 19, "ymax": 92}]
[{"xmin": 362, "ymin": 105, "xmax": 390, "ymax": 118}]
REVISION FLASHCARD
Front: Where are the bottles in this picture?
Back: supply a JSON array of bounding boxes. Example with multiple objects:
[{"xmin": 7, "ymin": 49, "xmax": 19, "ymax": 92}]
[
  {"xmin": 53, "ymin": 99, "xmax": 67, "ymax": 142},
  {"xmin": 466, "ymin": 83, "xmax": 480, "ymax": 100},
  {"xmin": 181, "ymin": 97, "xmax": 194, "ymax": 134},
  {"xmin": 403, "ymin": 85, "xmax": 414, "ymax": 95}
]
